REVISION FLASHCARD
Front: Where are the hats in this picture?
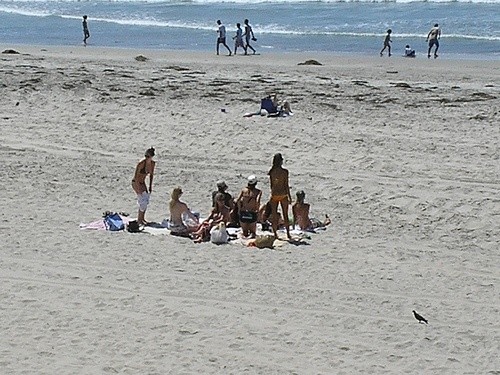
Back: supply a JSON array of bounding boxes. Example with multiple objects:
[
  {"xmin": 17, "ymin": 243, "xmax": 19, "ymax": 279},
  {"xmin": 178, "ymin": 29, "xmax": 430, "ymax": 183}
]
[
  {"xmin": 217, "ymin": 180, "xmax": 227, "ymax": 188},
  {"xmin": 248, "ymin": 175, "xmax": 258, "ymax": 184}
]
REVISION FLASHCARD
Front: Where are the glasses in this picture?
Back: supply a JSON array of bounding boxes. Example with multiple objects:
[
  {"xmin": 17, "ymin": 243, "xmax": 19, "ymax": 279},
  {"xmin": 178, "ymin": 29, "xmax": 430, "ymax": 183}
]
[{"xmin": 144, "ymin": 154, "xmax": 150, "ymax": 158}]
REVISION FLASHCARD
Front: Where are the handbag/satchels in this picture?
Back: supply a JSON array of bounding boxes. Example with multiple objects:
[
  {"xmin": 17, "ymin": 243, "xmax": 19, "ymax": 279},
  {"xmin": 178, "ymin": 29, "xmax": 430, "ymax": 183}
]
[
  {"xmin": 240, "ymin": 210, "xmax": 258, "ymax": 224},
  {"xmin": 253, "ymin": 231, "xmax": 276, "ymax": 248},
  {"xmin": 211, "ymin": 222, "xmax": 228, "ymax": 243},
  {"xmin": 101, "ymin": 210, "xmax": 124, "ymax": 231},
  {"xmin": 126, "ymin": 220, "xmax": 144, "ymax": 233}
]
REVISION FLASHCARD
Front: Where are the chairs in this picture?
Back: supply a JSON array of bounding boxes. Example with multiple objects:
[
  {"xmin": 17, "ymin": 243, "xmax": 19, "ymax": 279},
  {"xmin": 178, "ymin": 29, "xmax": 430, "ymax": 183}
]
[{"xmin": 261, "ymin": 97, "xmax": 289, "ymax": 117}]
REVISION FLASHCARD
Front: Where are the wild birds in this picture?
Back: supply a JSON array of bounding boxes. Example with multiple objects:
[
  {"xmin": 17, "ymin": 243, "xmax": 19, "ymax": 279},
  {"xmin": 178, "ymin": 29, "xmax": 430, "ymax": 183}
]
[{"xmin": 412, "ymin": 310, "xmax": 427, "ymax": 324}]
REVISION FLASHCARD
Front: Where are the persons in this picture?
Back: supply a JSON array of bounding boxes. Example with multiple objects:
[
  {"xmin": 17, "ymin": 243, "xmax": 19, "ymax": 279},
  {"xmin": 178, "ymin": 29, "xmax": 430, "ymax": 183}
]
[
  {"xmin": 405, "ymin": 45, "xmax": 415, "ymax": 57},
  {"xmin": 191, "ymin": 181, "xmax": 294, "ymax": 242},
  {"xmin": 132, "ymin": 147, "xmax": 155, "ymax": 225},
  {"xmin": 266, "ymin": 93, "xmax": 293, "ymax": 115},
  {"xmin": 169, "ymin": 189, "xmax": 201, "ymax": 230},
  {"xmin": 237, "ymin": 176, "xmax": 262, "ymax": 238},
  {"xmin": 380, "ymin": 29, "xmax": 392, "ymax": 56},
  {"xmin": 269, "ymin": 153, "xmax": 292, "ymax": 239},
  {"xmin": 241, "ymin": 19, "xmax": 256, "ymax": 55},
  {"xmin": 217, "ymin": 20, "xmax": 232, "ymax": 55},
  {"xmin": 232, "ymin": 23, "xmax": 247, "ymax": 54},
  {"xmin": 292, "ymin": 190, "xmax": 331, "ymax": 230},
  {"xmin": 82, "ymin": 15, "xmax": 90, "ymax": 43},
  {"xmin": 426, "ymin": 23, "xmax": 441, "ymax": 58}
]
